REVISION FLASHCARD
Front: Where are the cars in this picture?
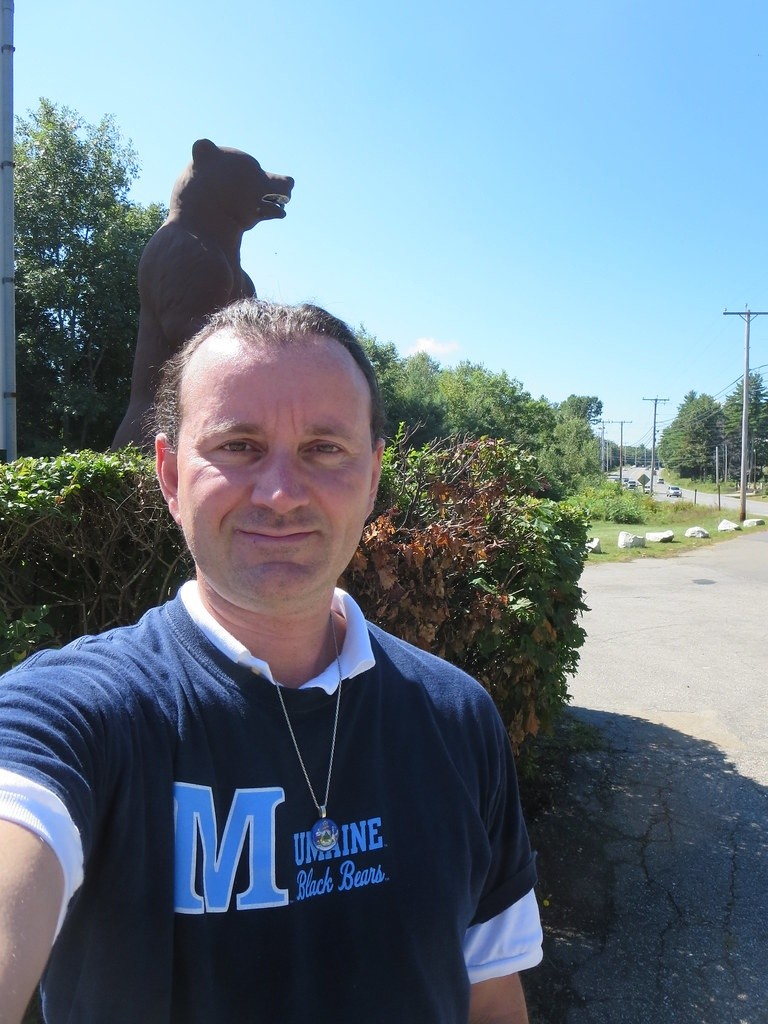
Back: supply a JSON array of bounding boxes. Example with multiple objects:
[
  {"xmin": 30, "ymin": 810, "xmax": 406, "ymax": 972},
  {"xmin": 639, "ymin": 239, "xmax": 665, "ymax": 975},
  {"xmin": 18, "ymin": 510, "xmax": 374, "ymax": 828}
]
[
  {"xmin": 644, "ymin": 485, "xmax": 650, "ymax": 493},
  {"xmin": 666, "ymin": 486, "xmax": 682, "ymax": 498},
  {"xmin": 627, "ymin": 480, "xmax": 638, "ymax": 489},
  {"xmin": 622, "ymin": 477, "xmax": 630, "ymax": 485},
  {"xmin": 656, "ymin": 478, "xmax": 664, "ymax": 484}
]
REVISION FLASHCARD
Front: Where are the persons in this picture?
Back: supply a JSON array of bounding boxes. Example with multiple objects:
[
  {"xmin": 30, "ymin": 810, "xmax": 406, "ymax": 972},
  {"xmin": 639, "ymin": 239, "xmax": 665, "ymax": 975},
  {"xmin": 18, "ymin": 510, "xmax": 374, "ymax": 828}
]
[{"xmin": 0, "ymin": 297, "xmax": 543, "ymax": 1024}]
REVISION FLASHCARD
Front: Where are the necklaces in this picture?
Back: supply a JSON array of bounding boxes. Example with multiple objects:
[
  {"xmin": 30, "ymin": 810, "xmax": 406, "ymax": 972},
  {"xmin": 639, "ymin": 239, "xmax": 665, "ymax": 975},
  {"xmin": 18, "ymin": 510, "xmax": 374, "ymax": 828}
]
[{"xmin": 274, "ymin": 611, "xmax": 343, "ymax": 852}]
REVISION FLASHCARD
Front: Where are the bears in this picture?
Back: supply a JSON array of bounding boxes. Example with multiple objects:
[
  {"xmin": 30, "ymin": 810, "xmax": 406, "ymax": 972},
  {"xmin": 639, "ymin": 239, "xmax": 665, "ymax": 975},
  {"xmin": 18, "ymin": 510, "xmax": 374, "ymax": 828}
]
[{"xmin": 105, "ymin": 139, "xmax": 294, "ymax": 453}]
